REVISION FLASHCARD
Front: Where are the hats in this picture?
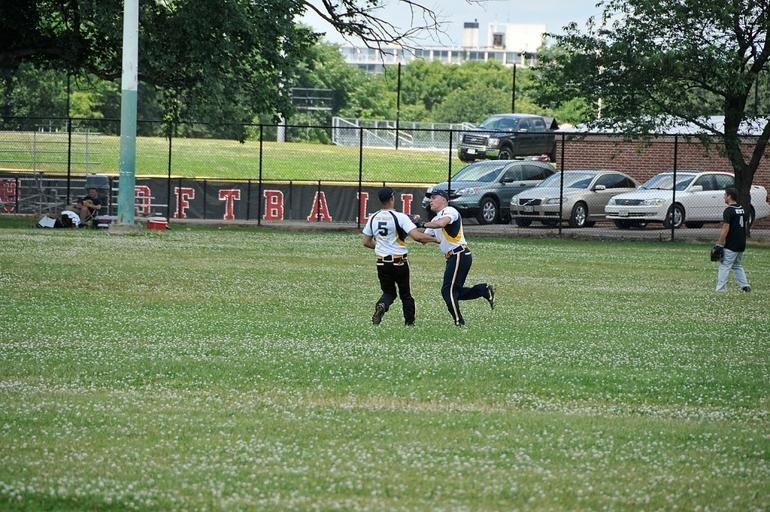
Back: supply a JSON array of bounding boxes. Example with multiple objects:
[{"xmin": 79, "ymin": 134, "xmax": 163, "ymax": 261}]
[
  {"xmin": 378, "ymin": 187, "xmax": 396, "ymax": 201},
  {"xmin": 425, "ymin": 190, "xmax": 449, "ymax": 201}
]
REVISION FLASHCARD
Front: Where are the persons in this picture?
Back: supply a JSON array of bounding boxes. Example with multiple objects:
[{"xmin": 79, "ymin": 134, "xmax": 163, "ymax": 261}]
[
  {"xmin": 411, "ymin": 188, "xmax": 496, "ymax": 326},
  {"xmin": 361, "ymin": 187, "xmax": 441, "ymax": 325},
  {"xmin": 63, "ymin": 188, "xmax": 103, "ymax": 225},
  {"xmin": 715, "ymin": 188, "xmax": 752, "ymax": 292}
]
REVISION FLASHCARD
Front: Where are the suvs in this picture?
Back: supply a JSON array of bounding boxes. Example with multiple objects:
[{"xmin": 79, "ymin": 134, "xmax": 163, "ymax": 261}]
[{"xmin": 422, "ymin": 159, "xmax": 559, "ymax": 227}]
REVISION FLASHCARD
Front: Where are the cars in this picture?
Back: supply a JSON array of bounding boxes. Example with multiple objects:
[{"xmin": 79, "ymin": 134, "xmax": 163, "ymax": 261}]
[
  {"xmin": 510, "ymin": 168, "xmax": 649, "ymax": 230},
  {"xmin": 602, "ymin": 169, "xmax": 770, "ymax": 230}
]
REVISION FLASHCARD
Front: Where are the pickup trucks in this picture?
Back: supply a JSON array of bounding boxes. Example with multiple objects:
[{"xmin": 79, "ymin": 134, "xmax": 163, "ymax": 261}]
[{"xmin": 456, "ymin": 112, "xmax": 558, "ymax": 164}]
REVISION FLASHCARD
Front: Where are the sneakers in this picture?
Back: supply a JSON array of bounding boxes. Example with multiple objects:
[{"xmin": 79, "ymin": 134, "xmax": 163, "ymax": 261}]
[
  {"xmin": 372, "ymin": 303, "xmax": 386, "ymax": 325},
  {"xmin": 486, "ymin": 285, "xmax": 496, "ymax": 311}
]
